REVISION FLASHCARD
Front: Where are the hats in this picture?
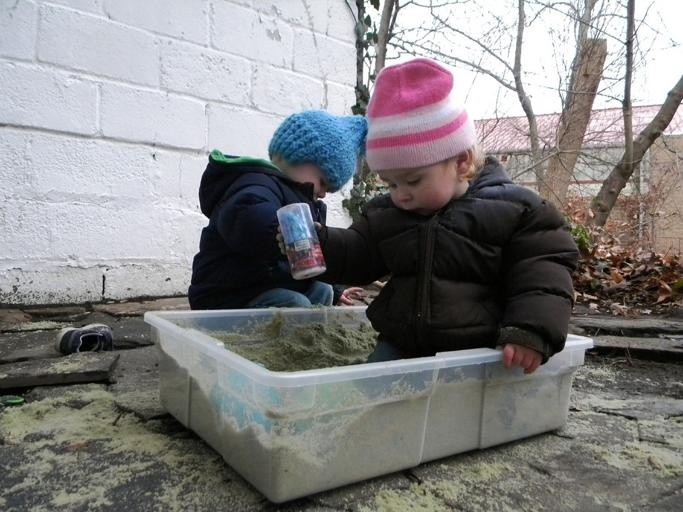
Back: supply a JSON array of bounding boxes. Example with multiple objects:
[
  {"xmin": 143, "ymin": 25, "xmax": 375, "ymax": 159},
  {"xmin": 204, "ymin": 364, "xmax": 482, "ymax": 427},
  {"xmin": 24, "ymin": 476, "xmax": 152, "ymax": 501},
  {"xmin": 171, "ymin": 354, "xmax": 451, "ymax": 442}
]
[
  {"xmin": 364, "ymin": 59, "xmax": 477, "ymax": 171},
  {"xmin": 267, "ymin": 110, "xmax": 367, "ymax": 192}
]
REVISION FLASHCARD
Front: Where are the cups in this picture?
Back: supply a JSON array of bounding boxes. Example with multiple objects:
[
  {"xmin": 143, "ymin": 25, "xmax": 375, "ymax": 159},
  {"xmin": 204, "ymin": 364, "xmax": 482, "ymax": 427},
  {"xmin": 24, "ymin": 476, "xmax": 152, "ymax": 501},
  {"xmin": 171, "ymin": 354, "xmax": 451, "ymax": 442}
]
[{"xmin": 275, "ymin": 202, "xmax": 327, "ymax": 281}]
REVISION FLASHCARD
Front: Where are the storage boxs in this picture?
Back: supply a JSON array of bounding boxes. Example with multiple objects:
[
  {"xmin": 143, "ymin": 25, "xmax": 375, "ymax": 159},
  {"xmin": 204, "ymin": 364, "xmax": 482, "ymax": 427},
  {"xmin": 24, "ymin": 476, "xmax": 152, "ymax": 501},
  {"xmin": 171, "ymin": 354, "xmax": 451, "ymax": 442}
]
[{"xmin": 144, "ymin": 306, "xmax": 595, "ymax": 503}]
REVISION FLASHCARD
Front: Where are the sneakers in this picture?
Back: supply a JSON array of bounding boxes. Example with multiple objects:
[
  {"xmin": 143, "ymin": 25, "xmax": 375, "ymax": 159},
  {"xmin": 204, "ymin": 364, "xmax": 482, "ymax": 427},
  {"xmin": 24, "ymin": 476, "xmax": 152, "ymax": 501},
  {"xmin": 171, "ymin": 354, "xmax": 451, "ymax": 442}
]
[{"xmin": 53, "ymin": 322, "xmax": 115, "ymax": 354}]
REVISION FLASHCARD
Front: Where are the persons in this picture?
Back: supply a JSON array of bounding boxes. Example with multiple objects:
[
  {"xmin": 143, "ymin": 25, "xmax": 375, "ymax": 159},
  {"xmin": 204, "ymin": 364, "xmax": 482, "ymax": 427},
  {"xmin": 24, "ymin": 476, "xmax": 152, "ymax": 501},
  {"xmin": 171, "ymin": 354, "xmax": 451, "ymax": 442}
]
[
  {"xmin": 187, "ymin": 110, "xmax": 368, "ymax": 332},
  {"xmin": 275, "ymin": 57, "xmax": 580, "ymax": 375}
]
